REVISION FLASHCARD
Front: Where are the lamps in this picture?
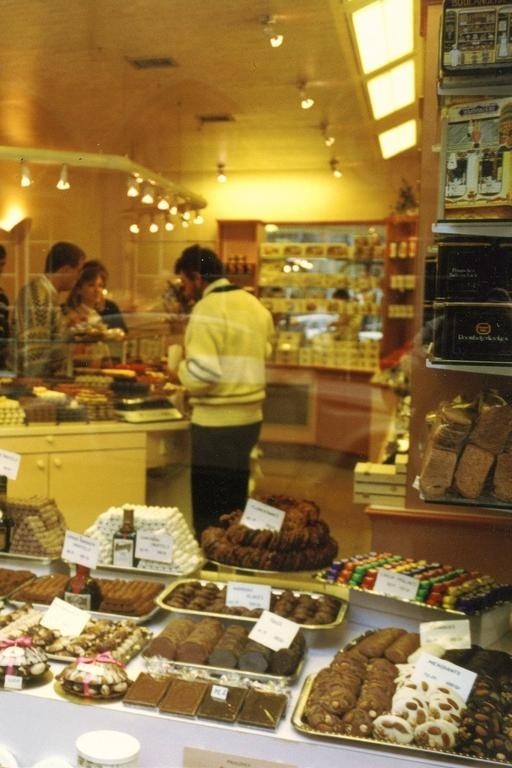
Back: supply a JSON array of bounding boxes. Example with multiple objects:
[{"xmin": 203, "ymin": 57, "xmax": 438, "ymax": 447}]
[{"xmin": 15, "ymin": 8, "xmax": 349, "ymax": 238}]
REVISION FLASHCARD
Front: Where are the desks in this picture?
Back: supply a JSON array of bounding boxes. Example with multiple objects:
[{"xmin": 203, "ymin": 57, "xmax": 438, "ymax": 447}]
[{"xmin": 0, "ymin": 541, "xmax": 511, "ymax": 768}]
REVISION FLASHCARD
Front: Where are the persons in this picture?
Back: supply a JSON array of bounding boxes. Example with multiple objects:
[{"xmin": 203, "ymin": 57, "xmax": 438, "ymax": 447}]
[
  {"xmin": 17, "ymin": 244, "xmax": 84, "ymax": 378},
  {"xmin": 169, "ymin": 245, "xmax": 275, "ymax": 540},
  {"xmin": 61, "ymin": 261, "xmax": 127, "ymax": 340},
  {"xmin": 329, "ymin": 291, "xmax": 354, "ymax": 345},
  {"xmin": 0, "ymin": 247, "xmax": 9, "ymax": 371}
]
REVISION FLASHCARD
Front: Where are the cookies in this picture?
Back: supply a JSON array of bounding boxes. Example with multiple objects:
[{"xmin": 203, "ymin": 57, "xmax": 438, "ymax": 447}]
[
  {"xmin": 0, "ymin": 367, "xmax": 177, "ymax": 427},
  {"xmin": 419, "ymin": 401, "xmax": 512, "ymax": 503}
]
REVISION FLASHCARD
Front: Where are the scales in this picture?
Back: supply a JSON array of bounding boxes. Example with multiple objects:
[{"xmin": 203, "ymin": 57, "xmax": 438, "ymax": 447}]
[{"xmin": 106, "ymin": 389, "xmax": 183, "ymax": 423}]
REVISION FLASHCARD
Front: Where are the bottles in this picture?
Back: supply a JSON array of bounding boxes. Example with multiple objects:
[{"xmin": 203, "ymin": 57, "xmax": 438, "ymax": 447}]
[{"xmin": 111, "ymin": 506, "xmax": 139, "ymax": 569}]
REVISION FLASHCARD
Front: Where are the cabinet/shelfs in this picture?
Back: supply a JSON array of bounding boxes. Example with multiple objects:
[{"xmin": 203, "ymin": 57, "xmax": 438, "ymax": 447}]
[
  {"xmin": 217, "ymin": 180, "xmax": 511, "ymax": 593},
  {"xmin": 0, "ymin": 417, "xmax": 148, "ymax": 544}
]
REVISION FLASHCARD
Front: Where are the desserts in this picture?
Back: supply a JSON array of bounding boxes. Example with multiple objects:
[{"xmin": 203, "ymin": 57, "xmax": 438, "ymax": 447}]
[{"xmin": 0, "ymin": 493, "xmax": 512, "ymax": 768}]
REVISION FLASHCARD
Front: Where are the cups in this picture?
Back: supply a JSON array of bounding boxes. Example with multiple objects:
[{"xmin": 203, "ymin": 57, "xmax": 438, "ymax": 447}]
[
  {"xmin": 167, "ymin": 343, "xmax": 184, "ymax": 373},
  {"xmin": 77, "ymin": 729, "xmax": 142, "ymax": 768}
]
